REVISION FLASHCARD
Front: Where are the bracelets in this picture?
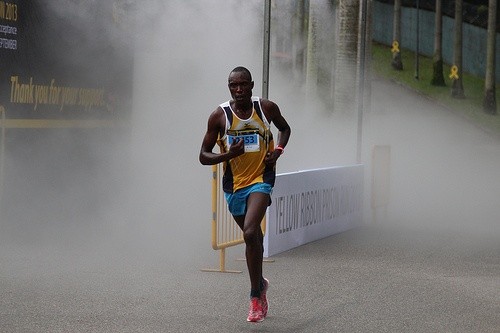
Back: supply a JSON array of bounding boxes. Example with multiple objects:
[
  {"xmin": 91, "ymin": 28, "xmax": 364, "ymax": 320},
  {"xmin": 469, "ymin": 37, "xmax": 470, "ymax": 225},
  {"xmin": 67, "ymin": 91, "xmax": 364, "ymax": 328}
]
[{"xmin": 275, "ymin": 145, "xmax": 283, "ymax": 153}]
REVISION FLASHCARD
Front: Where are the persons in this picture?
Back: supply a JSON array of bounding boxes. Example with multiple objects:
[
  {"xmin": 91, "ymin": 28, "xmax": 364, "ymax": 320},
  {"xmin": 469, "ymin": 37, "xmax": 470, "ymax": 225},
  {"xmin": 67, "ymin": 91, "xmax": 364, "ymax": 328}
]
[{"xmin": 199, "ymin": 66, "xmax": 291, "ymax": 323}]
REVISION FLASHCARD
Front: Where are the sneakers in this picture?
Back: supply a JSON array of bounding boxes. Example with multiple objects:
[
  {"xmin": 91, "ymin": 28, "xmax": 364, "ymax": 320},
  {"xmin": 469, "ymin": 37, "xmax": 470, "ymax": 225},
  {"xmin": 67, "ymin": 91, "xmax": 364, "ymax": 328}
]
[
  {"xmin": 261, "ymin": 277, "xmax": 270, "ymax": 317},
  {"xmin": 247, "ymin": 295, "xmax": 264, "ymax": 322}
]
